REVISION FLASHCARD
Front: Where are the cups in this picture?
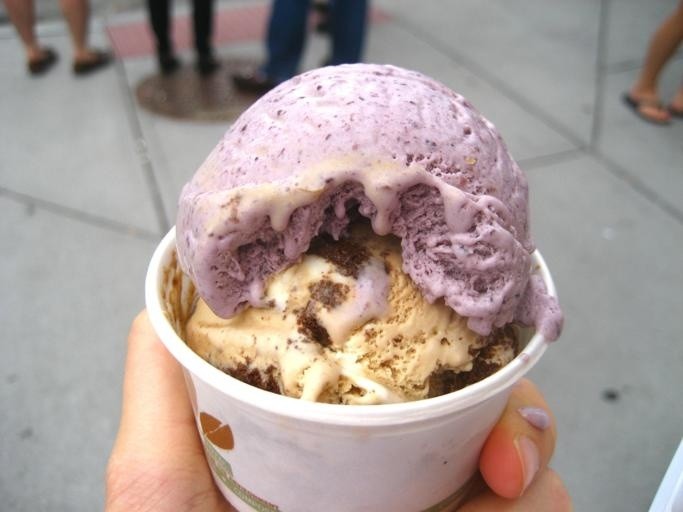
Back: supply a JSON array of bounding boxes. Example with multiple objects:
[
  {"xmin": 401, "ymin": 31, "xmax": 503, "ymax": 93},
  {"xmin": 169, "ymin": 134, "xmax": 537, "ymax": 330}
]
[{"xmin": 144, "ymin": 224, "xmax": 560, "ymax": 512}]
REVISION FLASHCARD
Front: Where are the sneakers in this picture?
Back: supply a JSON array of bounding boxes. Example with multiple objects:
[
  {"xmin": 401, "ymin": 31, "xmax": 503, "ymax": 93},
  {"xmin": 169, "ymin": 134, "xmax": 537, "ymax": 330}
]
[{"xmin": 227, "ymin": 67, "xmax": 276, "ymax": 97}]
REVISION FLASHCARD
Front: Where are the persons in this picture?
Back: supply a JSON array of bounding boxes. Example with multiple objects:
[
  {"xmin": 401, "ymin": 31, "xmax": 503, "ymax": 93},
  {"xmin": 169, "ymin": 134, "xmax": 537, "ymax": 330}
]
[
  {"xmin": 622, "ymin": 1, "xmax": 683, "ymax": 128},
  {"xmin": 104, "ymin": 307, "xmax": 574, "ymax": 511},
  {"xmin": 135, "ymin": 1, "xmax": 222, "ymax": 118},
  {"xmin": 229, "ymin": 0, "xmax": 367, "ymax": 99},
  {"xmin": 2, "ymin": 0, "xmax": 110, "ymax": 76}
]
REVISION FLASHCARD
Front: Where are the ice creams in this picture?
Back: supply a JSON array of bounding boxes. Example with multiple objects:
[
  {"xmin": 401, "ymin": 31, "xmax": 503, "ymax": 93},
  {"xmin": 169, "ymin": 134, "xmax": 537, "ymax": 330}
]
[{"xmin": 173, "ymin": 62, "xmax": 564, "ymax": 405}]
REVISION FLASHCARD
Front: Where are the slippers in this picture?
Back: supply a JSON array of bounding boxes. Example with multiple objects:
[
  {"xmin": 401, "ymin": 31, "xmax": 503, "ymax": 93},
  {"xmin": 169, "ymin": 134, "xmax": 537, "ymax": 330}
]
[{"xmin": 622, "ymin": 89, "xmax": 682, "ymax": 127}]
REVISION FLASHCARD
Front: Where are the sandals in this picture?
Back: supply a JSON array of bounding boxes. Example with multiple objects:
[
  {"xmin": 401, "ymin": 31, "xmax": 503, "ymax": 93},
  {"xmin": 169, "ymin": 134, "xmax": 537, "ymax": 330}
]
[{"xmin": 28, "ymin": 47, "xmax": 111, "ymax": 75}]
